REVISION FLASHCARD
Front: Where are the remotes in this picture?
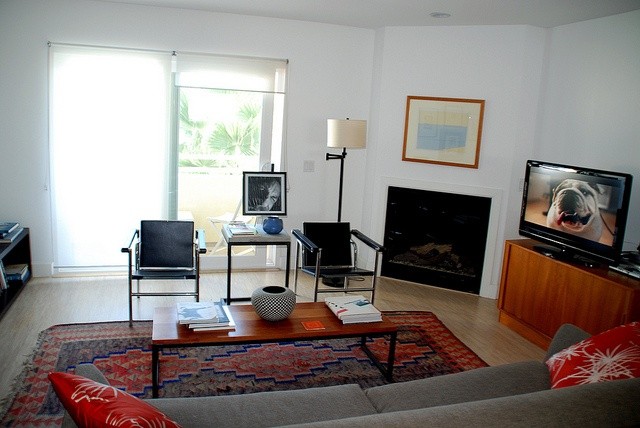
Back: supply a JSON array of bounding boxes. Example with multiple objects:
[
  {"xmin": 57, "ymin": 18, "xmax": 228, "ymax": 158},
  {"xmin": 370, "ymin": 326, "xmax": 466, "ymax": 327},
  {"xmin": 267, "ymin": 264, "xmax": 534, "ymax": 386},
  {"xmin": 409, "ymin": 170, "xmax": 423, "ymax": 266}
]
[{"xmin": 608, "ymin": 266, "xmax": 640, "ymax": 279}]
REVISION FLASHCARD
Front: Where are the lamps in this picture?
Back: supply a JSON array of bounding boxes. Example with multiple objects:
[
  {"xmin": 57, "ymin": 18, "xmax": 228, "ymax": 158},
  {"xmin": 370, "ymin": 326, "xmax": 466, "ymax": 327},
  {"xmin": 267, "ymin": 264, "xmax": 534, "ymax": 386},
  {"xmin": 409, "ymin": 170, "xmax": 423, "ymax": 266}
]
[{"xmin": 324, "ymin": 116, "xmax": 370, "ymax": 288}]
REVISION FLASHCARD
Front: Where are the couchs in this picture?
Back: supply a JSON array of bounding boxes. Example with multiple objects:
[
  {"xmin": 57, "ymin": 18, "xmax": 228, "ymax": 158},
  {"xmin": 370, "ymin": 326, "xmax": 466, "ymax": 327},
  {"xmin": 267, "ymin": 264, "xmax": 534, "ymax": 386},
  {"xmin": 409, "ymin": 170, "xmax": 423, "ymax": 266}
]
[{"xmin": 62, "ymin": 323, "xmax": 640, "ymax": 428}]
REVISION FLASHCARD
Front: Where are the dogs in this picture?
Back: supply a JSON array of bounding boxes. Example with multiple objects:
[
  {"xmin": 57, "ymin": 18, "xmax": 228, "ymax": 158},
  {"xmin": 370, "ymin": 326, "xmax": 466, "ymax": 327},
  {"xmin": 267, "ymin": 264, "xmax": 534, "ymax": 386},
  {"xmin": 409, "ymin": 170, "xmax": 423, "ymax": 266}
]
[{"xmin": 545, "ymin": 178, "xmax": 604, "ymax": 242}]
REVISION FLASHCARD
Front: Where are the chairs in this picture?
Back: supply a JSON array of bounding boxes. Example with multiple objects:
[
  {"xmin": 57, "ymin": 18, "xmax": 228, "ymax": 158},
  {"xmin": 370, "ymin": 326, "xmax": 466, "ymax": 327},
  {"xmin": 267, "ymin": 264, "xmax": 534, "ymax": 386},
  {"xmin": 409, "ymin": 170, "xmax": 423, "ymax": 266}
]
[
  {"xmin": 207, "ymin": 195, "xmax": 255, "ymax": 257},
  {"xmin": 120, "ymin": 220, "xmax": 208, "ymax": 329},
  {"xmin": 291, "ymin": 219, "xmax": 384, "ymax": 307}
]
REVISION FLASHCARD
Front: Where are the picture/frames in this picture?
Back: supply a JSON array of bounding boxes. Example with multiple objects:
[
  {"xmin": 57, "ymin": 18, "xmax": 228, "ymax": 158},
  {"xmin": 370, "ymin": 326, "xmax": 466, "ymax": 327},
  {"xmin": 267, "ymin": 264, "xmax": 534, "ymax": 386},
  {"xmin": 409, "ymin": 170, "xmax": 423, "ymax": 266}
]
[
  {"xmin": 240, "ymin": 170, "xmax": 288, "ymax": 217},
  {"xmin": 399, "ymin": 94, "xmax": 487, "ymax": 170}
]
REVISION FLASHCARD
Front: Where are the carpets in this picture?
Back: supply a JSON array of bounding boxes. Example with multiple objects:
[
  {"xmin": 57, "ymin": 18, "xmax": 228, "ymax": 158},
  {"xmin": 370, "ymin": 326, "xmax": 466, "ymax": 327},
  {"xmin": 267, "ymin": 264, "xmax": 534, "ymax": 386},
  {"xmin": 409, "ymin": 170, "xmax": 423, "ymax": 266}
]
[{"xmin": 1, "ymin": 306, "xmax": 491, "ymax": 428}]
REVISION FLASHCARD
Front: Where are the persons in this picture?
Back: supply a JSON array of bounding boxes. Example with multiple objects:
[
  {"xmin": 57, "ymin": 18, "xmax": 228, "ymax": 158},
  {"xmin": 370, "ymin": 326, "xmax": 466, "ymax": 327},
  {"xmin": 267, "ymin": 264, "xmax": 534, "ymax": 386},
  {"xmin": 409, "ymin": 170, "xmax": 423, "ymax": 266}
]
[{"xmin": 248, "ymin": 177, "xmax": 281, "ymax": 210}]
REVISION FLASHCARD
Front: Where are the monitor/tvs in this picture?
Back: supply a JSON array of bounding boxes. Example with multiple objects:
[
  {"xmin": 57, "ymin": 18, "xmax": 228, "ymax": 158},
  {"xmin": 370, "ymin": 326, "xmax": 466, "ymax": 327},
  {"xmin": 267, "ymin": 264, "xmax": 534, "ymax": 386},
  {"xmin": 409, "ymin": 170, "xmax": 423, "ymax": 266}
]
[{"xmin": 518, "ymin": 159, "xmax": 634, "ymax": 269}]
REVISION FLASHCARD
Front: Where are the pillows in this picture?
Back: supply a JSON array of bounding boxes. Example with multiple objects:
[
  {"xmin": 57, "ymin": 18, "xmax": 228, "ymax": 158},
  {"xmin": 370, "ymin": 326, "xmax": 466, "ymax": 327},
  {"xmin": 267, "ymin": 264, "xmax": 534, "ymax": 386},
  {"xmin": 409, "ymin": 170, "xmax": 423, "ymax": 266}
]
[
  {"xmin": 544, "ymin": 322, "xmax": 640, "ymax": 389},
  {"xmin": 48, "ymin": 368, "xmax": 180, "ymax": 428}
]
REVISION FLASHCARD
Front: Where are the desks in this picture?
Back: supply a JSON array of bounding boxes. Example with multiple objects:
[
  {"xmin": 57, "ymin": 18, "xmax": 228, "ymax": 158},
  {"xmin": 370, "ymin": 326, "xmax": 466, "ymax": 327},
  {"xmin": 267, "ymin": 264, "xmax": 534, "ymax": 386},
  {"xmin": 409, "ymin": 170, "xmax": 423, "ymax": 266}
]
[{"xmin": 216, "ymin": 223, "xmax": 292, "ymax": 307}]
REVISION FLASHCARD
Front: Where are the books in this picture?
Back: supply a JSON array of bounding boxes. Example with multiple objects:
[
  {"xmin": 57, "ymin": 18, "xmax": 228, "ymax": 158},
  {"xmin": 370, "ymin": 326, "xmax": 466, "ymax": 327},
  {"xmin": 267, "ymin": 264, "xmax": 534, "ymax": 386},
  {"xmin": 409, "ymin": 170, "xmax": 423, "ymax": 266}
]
[
  {"xmin": 228, "ymin": 220, "xmax": 252, "ymax": 235},
  {"xmin": 5, "ymin": 264, "xmax": 29, "ymax": 284},
  {"xmin": 178, "ymin": 301, "xmax": 236, "ymax": 332},
  {"xmin": 0, "ymin": 223, "xmax": 24, "ymax": 243},
  {"xmin": 326, "ymin": 294, "xmax": 383, "ymax": 325}
]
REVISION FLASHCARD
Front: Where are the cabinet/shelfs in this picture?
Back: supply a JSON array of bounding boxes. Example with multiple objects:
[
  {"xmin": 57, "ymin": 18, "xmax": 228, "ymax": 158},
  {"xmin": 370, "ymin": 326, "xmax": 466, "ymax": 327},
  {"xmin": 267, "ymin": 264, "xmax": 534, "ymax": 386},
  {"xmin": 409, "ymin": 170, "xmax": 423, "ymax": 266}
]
[
  {"xmin": 494, "ymin": 237, "xmax": 640, "ymax": 351},
  {"xmin": 0, "ymin": 226, "xmax": 34, "ymax": 322}
]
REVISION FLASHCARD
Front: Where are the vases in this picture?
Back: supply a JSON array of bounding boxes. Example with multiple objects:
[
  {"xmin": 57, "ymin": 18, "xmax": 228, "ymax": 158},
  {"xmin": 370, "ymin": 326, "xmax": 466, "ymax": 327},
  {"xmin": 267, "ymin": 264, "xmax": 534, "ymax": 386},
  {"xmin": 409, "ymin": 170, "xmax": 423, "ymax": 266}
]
[
  {"xmin": 250, "ymin": 285, "xmax": 297, "ymax": 322},
  {"xmin": 262, "ymin": 218, "xmax": 285, "ymax": 235}
]
